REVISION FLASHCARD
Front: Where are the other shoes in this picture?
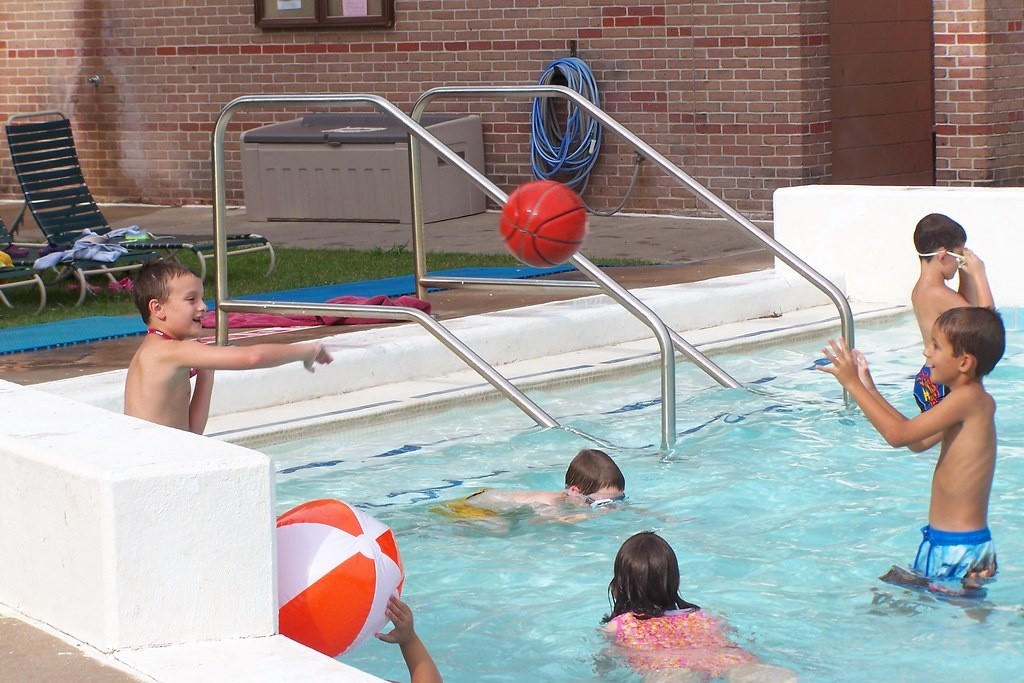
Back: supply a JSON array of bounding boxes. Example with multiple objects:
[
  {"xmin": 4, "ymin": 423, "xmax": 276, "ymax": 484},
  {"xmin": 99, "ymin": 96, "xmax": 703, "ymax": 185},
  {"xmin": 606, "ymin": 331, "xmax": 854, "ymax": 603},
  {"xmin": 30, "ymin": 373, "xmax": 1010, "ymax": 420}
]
[
  {"xmin": 1, "ymin": 244, "xmax": 30, "ymax": 258},
  {"xmin": 66, "ymin": 281, "xmax": 103, "ymax": 293},
  {"xmin": 107, "ymin": 277, "xmax": 133, "ymax": 291},
  {"xmin": 37, "ymin": 242, "xmax": 69, "ymax": 257}
]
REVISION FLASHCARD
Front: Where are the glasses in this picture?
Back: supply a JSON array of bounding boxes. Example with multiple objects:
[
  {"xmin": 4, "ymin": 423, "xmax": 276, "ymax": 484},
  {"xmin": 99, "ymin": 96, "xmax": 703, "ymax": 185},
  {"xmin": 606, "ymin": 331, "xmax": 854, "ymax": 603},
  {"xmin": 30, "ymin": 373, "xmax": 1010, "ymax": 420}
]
[
  {"xmin": 584, "ymin": 493, "xmax": 627, "ymax": 508},
  {"xmin": 946, "ymin": 251, "xmax": 966, "ymax": 269}
]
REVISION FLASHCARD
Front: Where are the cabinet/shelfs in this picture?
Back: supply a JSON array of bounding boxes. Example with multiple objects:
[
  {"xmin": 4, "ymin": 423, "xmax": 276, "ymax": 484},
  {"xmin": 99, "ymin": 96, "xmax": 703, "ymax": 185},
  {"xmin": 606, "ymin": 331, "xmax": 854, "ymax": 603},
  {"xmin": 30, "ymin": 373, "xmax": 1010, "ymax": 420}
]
[{"xmin": 240, "ymin": 111, "xmax": 488, "ymax": 226}]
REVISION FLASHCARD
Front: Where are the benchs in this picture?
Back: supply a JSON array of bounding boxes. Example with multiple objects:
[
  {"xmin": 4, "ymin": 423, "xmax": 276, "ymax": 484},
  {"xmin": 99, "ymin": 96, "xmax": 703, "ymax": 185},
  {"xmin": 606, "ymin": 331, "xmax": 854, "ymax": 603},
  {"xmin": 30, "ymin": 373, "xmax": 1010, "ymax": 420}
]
[{"xmin": 0, "ymin": 111, "xmax": 276, "ymax": 317}]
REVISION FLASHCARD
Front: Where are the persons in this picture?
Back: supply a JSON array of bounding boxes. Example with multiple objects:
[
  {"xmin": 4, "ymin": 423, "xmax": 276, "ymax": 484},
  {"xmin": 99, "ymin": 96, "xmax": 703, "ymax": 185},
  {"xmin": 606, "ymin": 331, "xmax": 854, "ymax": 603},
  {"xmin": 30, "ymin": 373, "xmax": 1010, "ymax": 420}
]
[
  {"xmin": 605, "ymin": 529, "xmax": 712, "ymax": 649},
  {"xmin": 814, "ymin": 306, "xmax": 1007, "ymax": 578},
  {"xmin": 564, "ymin": 448, "xmax": 626, "ymax": 501},
  {"xmin": 373, "ymin": 593, "xmax": 443, "ymax": 682},
  {"xmin": 909, "ymin": 212, "xmax": 995, "ymax": 414},
  {"xmin": 124, "ymin": 258, "xmax": 333, "ymax": 435}
]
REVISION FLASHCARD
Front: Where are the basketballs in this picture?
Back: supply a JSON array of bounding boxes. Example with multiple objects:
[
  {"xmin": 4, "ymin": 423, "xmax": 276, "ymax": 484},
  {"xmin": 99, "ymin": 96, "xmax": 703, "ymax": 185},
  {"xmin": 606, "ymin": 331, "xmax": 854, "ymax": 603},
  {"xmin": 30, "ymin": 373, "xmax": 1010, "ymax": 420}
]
[{"xmin": 500, "ymin": 180, "xmax": 588, "ymax": 268}]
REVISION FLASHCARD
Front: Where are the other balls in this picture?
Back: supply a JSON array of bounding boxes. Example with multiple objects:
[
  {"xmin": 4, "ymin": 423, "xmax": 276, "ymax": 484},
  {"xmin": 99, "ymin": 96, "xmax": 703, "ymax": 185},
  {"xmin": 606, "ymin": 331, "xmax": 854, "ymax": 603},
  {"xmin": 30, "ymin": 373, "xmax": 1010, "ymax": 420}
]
[{"xmin": 277, "ymin": 498, "xmax": 405, "ymax": 656}]
